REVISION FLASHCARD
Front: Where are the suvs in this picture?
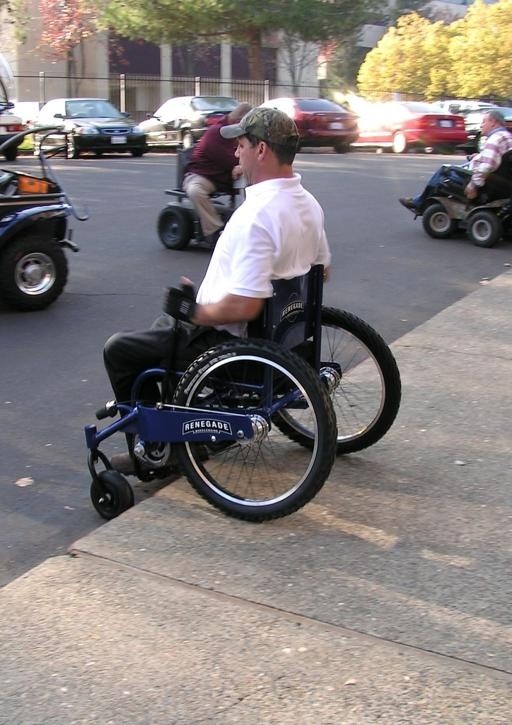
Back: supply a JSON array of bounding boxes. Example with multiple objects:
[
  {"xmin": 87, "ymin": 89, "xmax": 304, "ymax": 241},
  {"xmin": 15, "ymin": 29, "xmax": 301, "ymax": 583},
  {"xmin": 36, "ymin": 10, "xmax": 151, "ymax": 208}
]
[{"xmin": 0, "ymin": 125, "xmax": 83, "ymax": 310}]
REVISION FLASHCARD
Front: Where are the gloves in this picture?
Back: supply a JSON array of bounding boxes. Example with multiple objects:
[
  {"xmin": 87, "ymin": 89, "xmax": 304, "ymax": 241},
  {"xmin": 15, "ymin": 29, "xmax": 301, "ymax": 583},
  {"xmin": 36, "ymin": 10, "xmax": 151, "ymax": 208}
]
[{"xmin": 163, "ymin": 285, "xmax": 198, "ymax": 323}]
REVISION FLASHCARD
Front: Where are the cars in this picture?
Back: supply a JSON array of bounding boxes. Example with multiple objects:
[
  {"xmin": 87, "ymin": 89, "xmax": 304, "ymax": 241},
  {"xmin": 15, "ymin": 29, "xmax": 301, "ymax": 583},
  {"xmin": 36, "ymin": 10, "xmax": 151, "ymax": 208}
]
[
  {"xmin": 33, "ymin": 98, "xmax": 148, "ymax": 159},
  {"xmin": 257, "ymin": 95, "xmax": 360, "ymax": 154},
  {"xmin": 141, "ymin": 95, "xmax": 242, "ymax": 148},
  {"xmin": 459, "ymin": 105, "xmax": 512, "ymax": 153},
  {"xmin": 350, "ymin": 100, "xmax": 467, "ymax": 153}
]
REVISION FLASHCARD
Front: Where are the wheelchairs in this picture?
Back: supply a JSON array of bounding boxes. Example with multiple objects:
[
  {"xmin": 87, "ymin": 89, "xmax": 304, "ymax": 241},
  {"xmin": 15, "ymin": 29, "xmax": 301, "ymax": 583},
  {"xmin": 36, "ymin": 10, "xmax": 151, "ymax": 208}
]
[{"xmin": 86, "ymin": 263, "xmax": 401, "ymax": 525}]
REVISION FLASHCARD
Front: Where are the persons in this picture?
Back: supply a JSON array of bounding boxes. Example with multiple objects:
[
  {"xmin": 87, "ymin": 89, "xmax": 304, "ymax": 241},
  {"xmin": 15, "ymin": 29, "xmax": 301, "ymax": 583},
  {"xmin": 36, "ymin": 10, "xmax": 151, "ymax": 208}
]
[
  {"xmin": 103, "ymin": 107, "xmax": 331, "ymax": 477},
  {"xmin": 182, "ymin": 101, "xmax": 253, "ymax": 244},
  {"xmin": 398, "ymin": 111, "xmax": 512, "ymax": 216}
]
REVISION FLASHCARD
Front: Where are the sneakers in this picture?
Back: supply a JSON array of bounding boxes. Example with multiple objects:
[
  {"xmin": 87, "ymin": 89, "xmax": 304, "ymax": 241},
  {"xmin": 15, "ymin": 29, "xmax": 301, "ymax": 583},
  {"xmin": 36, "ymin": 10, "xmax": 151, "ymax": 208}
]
[
  {"xmin": 399, "ymin": 196, "xmax": 416, "ymax": 208},
  {"xmin": 111, "ymin": 452, "xmax": 137, "ymax": 476}
]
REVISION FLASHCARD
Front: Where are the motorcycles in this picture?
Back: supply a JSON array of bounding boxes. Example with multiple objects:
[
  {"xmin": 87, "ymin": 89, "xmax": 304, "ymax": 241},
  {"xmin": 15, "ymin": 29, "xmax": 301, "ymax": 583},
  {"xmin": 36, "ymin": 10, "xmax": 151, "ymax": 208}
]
[
  {"xmin": 417, "ymin": 148, "xmax": 510, "ymax": 246},
  {"xmin": 156, "ymin": 140, "xmax": 242, "ymax": 251}
]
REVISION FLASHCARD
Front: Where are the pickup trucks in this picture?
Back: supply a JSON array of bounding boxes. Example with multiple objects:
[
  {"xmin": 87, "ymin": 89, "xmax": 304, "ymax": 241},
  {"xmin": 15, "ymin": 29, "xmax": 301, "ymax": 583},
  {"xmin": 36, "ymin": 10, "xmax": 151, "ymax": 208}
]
[{"xmin": 0, "ymin": 72, "xmax": 28, "ymax": 163}]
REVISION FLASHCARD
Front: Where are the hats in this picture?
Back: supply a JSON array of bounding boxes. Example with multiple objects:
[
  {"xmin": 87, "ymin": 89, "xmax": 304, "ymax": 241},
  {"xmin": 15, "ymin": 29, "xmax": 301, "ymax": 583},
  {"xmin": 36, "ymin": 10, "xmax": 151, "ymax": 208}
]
[{"xmin": 219, "ymin": 106, "xmax": 301, "ymax": 150}]
